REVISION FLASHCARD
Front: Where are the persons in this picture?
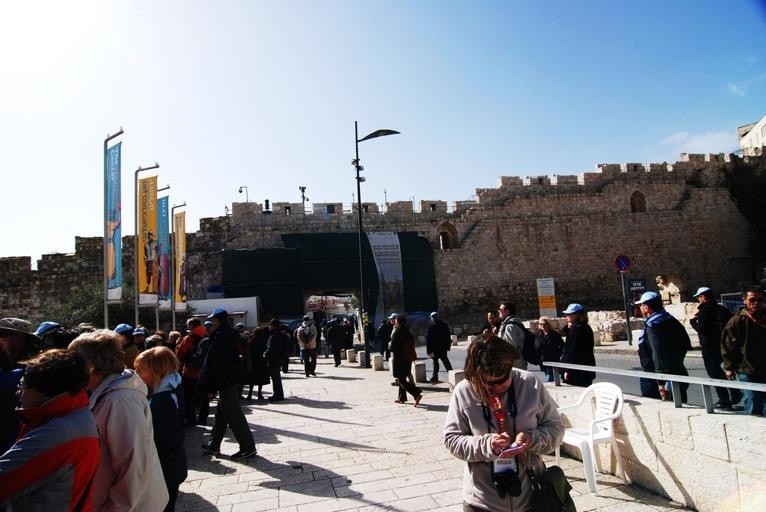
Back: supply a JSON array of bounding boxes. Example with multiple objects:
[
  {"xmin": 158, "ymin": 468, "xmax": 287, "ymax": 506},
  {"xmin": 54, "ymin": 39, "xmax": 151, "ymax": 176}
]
[
  {"xmin": 366, "ymin": 312, "xmax": 423, "ymax": 406},
  {"xmin": 245, "ymin": 314, "xmax": 353, "ymax": 403},
  {"xmin": 485, "ymin": 301, "xmax": 596, "ymax": 387},
  {"xmin": 0, "ymin": 309, "xmax": 257, "ymax": 512},
  {"xmin": 443, "ymin": 333, "xmax": 563, "ymax": 512},
  {"xmin": 426, "ymin": 311, "xmax": 453, "ymax": 382},
  {"xmin": 634, "ymin": 287, "xmax": 766, "ymax": 416}
]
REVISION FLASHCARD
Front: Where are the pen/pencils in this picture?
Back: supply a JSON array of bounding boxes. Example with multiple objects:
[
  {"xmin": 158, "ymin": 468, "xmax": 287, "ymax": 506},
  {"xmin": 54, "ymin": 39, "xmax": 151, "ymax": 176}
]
[{"xmin": 497, "ymin": 431, "xmax": 514, "ymax": 442}]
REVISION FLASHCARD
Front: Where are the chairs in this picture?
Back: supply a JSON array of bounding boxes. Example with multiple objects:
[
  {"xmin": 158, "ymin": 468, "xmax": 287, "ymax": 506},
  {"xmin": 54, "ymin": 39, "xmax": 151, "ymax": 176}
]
[{"xmin": 551, "ymin": 381, "xmax": 629, "ymax": 493}]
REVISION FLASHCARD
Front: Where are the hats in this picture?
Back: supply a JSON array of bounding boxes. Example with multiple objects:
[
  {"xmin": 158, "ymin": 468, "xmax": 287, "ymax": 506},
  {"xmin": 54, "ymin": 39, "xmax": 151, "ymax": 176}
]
[
  {"xmin": 693, "ymin": 287, "xmax": 710, "ymax": 297},
  {"xmin": 388, "ymin": 313, "xmax": 399, "ymax": 319},
  {"xmin": 635, "ymin": 291, "xmax": 657, "ymax": 305},
  {"xmin": 33, "ymin": 321, "xmax": 59, "ymax": 334},
  {"xmin": 1, "ymin": 317, "xmax": 42, "ymax": 340},
  {"xmin": 114, "ymin": 324, "xmax": 132, "ymax": 333},
  {"xmin": 133, "ymin": 326, "xmax": 145, "ymax": 335},
  {"xmin": 562, "ymin": 304, "xmax": 582, "ymax": 314},
  {"xmin": 428, "ymin": 312, "xmax": 439, "ymax": 317},
  {"xmin": 207, "ymin": 307, "xmax": 225, "ymax": 319}
]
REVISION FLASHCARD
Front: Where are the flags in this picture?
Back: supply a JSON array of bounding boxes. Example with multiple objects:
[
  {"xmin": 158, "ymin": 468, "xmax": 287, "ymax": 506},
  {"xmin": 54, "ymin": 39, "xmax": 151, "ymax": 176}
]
[
  {"xmin": 357, "ymin": 129, "xmax": 400, "ymax": 142},
  {"xmin": 105, "ymin": 141, "xmax": 189, "ymax": 305}
]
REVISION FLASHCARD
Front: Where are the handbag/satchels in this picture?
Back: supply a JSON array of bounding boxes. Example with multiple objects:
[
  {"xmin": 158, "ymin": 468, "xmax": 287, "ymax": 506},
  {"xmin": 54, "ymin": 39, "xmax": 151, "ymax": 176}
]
[
  {"xmin": 720, "ymin": 346, "xmax": 754, "ymax": 381},
  {"xmin": 409, "ymin": 342, "xmax": 417, "ymax": 362},
  {"xmin": 531, "ymin": 465, "xmax": 576, "ymax": 511}
]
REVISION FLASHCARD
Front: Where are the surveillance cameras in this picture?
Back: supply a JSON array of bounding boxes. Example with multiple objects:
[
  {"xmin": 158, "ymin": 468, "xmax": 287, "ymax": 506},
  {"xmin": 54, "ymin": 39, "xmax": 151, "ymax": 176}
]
[{"xmin": 239, "ymin": 187, "xmax": 243, "ymax": 194}]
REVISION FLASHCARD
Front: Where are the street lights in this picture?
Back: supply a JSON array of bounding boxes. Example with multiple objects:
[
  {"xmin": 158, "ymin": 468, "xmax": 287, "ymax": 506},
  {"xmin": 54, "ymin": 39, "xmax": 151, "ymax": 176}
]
[
  {"xmin": 239, "ymin": 186, "xmax": 248, "ymax": 203},
  {"xmin": 352, "ymin": 121, "xmax": 401, "ymax": 371}
]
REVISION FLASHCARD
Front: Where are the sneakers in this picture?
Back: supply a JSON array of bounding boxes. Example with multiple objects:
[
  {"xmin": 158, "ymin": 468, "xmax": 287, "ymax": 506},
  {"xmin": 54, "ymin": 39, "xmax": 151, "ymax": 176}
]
[
  {"xmin": 414, "ymin": 395, "xmax": 422, "ymax": 407},
  {"xmin": 269, "ymin": 396, "xmax": 284, "ymax": 400},
  {"xmin": 731, "ymin": 393, "xmax": 743, "ymax": 405},
  {"xmin": 395, "ymin": 400, "xmax": 405, "ymax": 404},
  {"xmin": 231, "ymin": 448, "xmax": 257, "ymax": 460},
  {"xmin": 201, "ymin": 445, "xmax": 220, "ymax": 455},
  {"xmin": 713, "ymin": 402, "xmax": 731, "ymax": 410},
  {"xmin": 246, "ymin": 397, "xmax": 251, "ymax": 400},
  {"xmin": 258, "ymin": 396, "xmax": 263, "ymax": 399}
]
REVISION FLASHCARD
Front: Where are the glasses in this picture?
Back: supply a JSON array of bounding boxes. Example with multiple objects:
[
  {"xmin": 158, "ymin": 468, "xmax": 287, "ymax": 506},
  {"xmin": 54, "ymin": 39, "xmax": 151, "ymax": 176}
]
[
  {"xmin": 540, "ymin": 323, "xmax": 546, "ymax": 325},
  {"xmin": 746, "ymin": 298, "xmax": 755, "ymax": 302},
  {"xmin": 17, "ymin": 383, "xmax": 28, "ymax": 388},
  {"xmin": 483, "ymin": 373, "xmax": 510, "ymax": 385}
]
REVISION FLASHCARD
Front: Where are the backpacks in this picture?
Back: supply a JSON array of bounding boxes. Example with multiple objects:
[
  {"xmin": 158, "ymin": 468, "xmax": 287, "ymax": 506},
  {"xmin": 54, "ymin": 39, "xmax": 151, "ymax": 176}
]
[
  {"xmin": 189, "ymin": 333, "xmax": 209, "ymax": 368},
  {"xmin": 299, "ymin": 323, "xmax": 314, "ymax": 344},
  {"xmin": 506, "ymin": 321, "xmax": 543, "ymax": 365}
]
